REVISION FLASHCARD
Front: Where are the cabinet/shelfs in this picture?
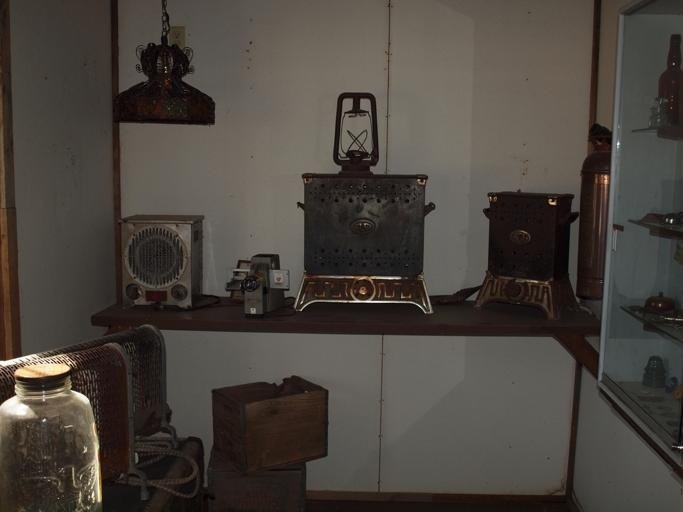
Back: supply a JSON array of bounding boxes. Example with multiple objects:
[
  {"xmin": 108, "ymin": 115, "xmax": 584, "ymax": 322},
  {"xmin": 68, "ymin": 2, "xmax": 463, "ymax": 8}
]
[
  {"xmin": 573, "ymin": 367, "xmax": 683, "ymax": 507},
  {"xmin": 595, "ymin": 0, "xmax": 680, "ymax": 472},
  {"xmin": 158, "ymin": 329, "xmax": 578, "ymax": 496}
]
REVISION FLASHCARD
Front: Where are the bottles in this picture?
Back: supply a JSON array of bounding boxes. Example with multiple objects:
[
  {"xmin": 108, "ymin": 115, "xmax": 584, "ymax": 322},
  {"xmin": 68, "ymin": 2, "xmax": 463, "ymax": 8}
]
[
  {"xmin": 658, "ymin": 34, "xmax": 682, "ymax": 140},
  {"xmin": 0, "ymin": 364, "xmax": 103, "ymax": 511}
]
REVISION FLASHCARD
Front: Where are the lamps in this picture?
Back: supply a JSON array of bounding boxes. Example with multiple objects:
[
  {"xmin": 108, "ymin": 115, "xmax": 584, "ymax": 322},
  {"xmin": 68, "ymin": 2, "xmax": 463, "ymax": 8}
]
[{"xmin": 112, "ymin": 0, "xmax": 215, "ymax": 126}]
